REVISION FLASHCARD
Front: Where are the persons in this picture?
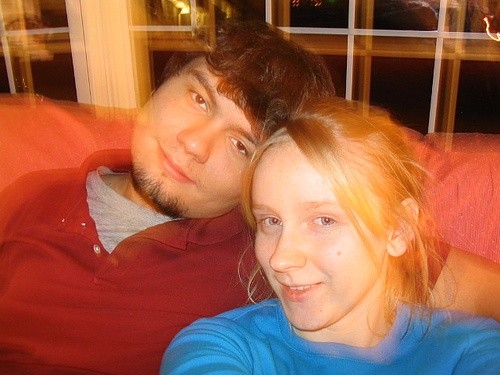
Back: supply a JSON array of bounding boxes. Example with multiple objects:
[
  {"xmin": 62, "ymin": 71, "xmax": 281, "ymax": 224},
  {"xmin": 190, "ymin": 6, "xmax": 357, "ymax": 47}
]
[
  {"xmin": 0, "ymin": 16, "xmax": 336, "ymax": 375},
  {"xmin": 160, "ymin": 93, "xmax": 500, "ymax": 375}
]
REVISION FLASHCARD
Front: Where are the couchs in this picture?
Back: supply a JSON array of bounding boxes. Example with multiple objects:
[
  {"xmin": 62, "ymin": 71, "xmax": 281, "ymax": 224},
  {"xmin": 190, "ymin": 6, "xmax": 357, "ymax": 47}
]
[{"xmin": 0, "ymin": 92, "xmax": 500, "ymax": 268}]
